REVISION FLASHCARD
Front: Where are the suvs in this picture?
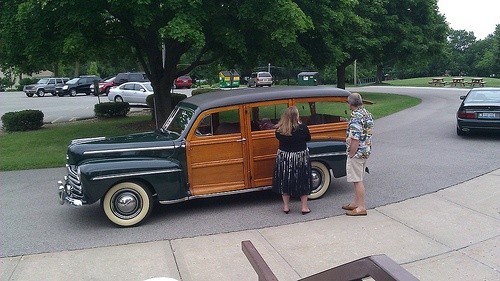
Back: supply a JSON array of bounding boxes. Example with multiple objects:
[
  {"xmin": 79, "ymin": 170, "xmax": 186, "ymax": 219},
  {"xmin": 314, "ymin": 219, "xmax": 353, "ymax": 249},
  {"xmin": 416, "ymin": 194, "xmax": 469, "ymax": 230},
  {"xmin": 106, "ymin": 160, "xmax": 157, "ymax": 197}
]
[
  {"xmin": 112, "ymin": 73, "xmax": 151, "ymax": 87},
  {"xmin": 23, "ymin": 78, "xmax": 70, "ymax": 97},
  {"xmin": 54, "ymin": 75, "xmax": 103, "ymax": 98},
  {"xmin": 60, "ymin": 86, "xmax": 375, "ymax": 229}
]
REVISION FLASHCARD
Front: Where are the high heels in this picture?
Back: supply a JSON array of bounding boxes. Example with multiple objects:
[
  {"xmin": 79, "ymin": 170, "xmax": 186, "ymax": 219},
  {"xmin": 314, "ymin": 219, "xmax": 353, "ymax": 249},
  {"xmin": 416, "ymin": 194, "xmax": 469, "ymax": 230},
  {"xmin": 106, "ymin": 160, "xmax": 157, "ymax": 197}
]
[
  {"xmin": 302, "ymin": 209, "xmax": 311, "ymax": 215},
  {"xmin": 284, "ymin": 209, "xmax": 290, "ymax": 214}
]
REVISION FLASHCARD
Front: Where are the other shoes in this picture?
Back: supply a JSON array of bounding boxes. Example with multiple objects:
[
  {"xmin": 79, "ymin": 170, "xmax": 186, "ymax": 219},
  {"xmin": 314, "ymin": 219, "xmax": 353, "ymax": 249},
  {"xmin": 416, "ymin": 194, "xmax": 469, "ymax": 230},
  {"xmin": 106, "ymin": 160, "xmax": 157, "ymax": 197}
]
[
  {"xmin": 342, "ymin": 203, "xmax": 357, "ymax": 210},
  {"xmin": 346, "ymin": 208, "xmax": 367, "ymax": 215}
]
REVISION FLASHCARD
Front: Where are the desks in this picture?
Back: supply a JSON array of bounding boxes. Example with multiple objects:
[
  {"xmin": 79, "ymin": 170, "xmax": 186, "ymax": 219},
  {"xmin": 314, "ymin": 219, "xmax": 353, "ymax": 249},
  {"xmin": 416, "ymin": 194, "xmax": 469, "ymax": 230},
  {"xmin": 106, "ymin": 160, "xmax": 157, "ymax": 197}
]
[
  {"xmin": 432, "ymin": 78, "xmax": 443, "ymax": 86},
  {"xmin": 451, "ymin": 78, "xmax": 465, "ymax": 86},
  {"xmin": 470, "ymin": 78, "xmax": 483, "ymax": 87}
]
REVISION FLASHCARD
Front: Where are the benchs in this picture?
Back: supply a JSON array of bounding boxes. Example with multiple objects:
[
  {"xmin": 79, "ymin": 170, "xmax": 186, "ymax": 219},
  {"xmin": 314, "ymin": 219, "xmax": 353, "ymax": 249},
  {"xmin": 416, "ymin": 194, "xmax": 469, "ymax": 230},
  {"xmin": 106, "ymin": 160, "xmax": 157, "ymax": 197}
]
[{"xmin": 428, "ymin": 81, "xmax": 486, "ymax": 88}]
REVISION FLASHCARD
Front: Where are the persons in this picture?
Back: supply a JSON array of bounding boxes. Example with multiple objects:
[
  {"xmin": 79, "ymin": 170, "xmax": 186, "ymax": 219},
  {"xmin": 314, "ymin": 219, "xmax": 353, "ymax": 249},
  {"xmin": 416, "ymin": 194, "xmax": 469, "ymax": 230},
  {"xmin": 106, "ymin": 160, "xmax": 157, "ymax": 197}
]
[
  {"xmin": 341, "ymin": 92, "xmax": 374, "ymax": 215},
  {"xmin": 272, "ymin": 106, "xmax": 312, "ymax": 215}
]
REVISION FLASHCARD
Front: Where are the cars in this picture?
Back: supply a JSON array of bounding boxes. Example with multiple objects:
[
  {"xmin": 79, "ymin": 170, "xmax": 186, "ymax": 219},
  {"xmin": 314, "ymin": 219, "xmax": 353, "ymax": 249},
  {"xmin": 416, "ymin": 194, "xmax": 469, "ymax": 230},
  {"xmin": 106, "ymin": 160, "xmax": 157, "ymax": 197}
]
[
  {"xmin": 247, "ymin": 72, "xmax": 273, "ymax": 88},
  {"xmin": 90, "ymin": 76, "xmax": 116, "ymax": 96},
  {"xmin": 107, "ymin": 82, "xmax": 154, "ymax": 106},
  {"xmin": 456, "ymin": 87, "xmax": 500, "ymax": 136},
  {"xmin": 174, "ymin": 74, "xmax": 192, "ymax": 89}
]
